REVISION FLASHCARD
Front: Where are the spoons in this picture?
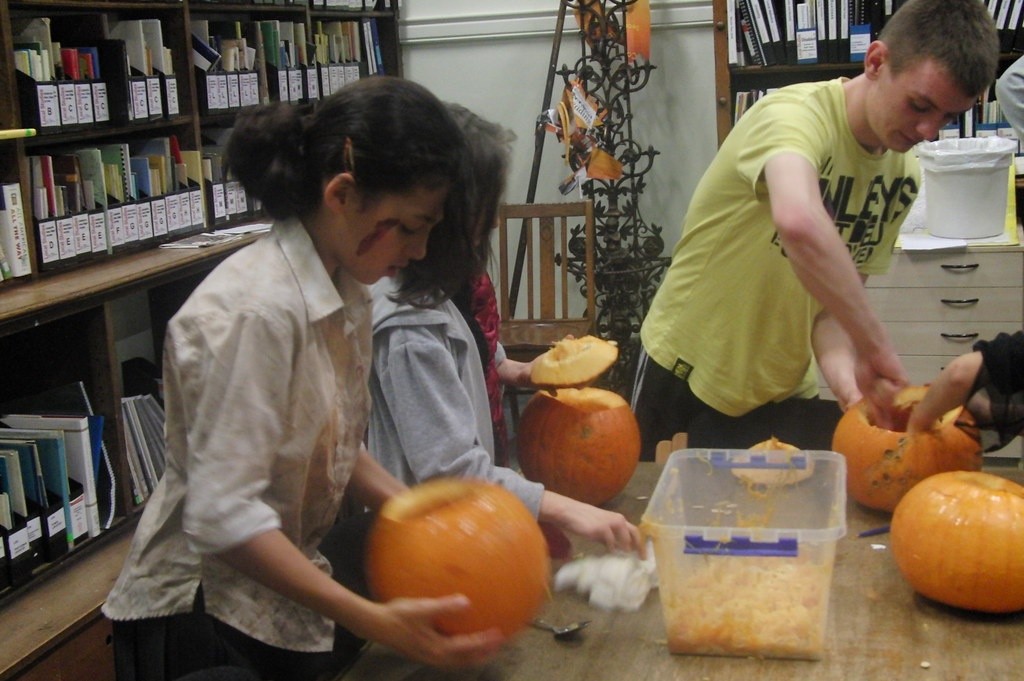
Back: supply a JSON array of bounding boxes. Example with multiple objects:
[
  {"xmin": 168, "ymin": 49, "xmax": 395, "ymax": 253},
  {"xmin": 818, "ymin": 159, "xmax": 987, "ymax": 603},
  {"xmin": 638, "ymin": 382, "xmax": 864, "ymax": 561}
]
[{"xmin": 529, "ymin": 618, "xmax": 593, "ymax": 639}]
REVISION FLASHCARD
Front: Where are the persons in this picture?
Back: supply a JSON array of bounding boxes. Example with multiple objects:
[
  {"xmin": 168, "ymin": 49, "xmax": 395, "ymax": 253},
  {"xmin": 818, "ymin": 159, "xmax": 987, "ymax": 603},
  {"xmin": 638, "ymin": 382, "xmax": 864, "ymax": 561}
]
[
  {"xmin": 100, "ymin": 73, "xmax": 502, "ymax": 681},
  {"xmin": 627, "ymin": 0, "xmax": 1000, "ymax": 461},
  {"xmin": 906, "ymin": 331, "xmax": 1024, "ymax": 453},
  {"xmin": 360, "ymin": 103, "xmax": 648, "ymax": 560},
  {"xmin": 994, "ymin": 55, "xmax": 1024, "ymax": 143}
]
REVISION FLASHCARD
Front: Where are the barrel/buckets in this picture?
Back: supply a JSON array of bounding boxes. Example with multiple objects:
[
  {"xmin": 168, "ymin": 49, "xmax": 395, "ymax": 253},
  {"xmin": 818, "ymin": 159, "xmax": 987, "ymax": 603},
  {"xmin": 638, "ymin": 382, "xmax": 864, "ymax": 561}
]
[{"xmin": 913, "ymin": 138, "xmax": 1017, "ymax": 239}]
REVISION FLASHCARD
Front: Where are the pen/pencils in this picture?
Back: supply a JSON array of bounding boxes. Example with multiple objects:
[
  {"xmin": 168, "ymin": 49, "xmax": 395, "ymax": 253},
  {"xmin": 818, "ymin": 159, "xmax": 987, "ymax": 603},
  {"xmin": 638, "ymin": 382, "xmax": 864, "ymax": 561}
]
[{"xmin": 856, "ymin": 525, "xmax": 891, "ymax": 538}]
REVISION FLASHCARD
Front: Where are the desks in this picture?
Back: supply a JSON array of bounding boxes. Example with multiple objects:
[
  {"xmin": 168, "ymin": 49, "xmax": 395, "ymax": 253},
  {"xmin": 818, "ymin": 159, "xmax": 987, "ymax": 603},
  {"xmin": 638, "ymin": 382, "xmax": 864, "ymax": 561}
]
[{"xmin": 328, "ymin": 459, "xmax": 1024, "ymax": 681}]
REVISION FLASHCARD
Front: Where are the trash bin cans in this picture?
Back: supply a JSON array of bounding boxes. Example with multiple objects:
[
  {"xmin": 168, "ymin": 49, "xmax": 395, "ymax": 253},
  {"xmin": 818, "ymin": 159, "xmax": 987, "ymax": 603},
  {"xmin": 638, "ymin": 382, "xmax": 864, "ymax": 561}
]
[{"xmin": 916, "ymin": 135, "xmax": 1018, "ymax": 239}]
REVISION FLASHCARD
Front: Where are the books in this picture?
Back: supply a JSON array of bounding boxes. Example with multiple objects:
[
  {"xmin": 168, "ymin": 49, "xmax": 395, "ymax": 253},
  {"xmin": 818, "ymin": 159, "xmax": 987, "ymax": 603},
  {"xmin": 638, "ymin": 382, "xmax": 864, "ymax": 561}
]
[
  {"xmin": 120, "ymin": 394, "xmax": 165, "ymax": 505},
  {"xmin": 0, "ymin": 414, "xmax": 100, "ymax": 537},
  {"xmin": 14, "ymin": 21, "xmax": 360, "ymax": 81},
  {"xmin": 727, "ymin": 0, "xmax": 1024, "ymax": 125},
  {"xmin": 25, "ymin": 135, "xmax": 232, "ymax": 225}
]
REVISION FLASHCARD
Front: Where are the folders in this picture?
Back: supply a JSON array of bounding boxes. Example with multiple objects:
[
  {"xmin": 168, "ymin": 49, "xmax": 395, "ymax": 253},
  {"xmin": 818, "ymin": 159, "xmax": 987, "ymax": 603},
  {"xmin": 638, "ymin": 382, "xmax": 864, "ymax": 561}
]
[{"xmin": 745, "ymin": 0, "xmax": 1024, "ymax": 66}]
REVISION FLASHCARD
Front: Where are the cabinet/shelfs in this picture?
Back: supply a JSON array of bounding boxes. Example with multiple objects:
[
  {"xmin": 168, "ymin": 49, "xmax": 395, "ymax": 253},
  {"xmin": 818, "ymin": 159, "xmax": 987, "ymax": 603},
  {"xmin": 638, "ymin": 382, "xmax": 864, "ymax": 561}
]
[
  {"xmin": 0, "ymin": 0, "xmax": 403, "ymax": 681},
  {"xmin": 817, "ymin": 222, "xmax": 1024, "ymax": 401},
  {"xmin": 712, "ymin": 0, "xmax": 1024, "ymax": 200}
]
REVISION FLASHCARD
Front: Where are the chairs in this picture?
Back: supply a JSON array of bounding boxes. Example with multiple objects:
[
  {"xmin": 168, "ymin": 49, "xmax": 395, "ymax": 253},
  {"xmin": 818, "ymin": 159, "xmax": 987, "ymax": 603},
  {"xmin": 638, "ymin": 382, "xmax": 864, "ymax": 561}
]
[{"xmin": 494, "ymin": 198, "xmax": 601, "ymax": 434}]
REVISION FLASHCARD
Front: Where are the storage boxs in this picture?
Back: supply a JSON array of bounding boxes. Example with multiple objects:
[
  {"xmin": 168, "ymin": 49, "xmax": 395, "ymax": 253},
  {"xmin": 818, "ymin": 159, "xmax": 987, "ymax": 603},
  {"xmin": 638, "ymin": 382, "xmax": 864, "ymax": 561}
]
[{"xmin": 639, "ymin": 448, "xmax": 848, "ymax": 663}]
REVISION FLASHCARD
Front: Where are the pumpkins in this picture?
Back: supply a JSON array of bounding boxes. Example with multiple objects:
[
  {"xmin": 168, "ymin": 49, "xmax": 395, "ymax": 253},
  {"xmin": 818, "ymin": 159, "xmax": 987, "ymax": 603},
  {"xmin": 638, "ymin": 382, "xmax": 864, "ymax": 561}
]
[{"xmin": 889, "ymin": 470, "xmax": 1024, "ymax": 614}]
